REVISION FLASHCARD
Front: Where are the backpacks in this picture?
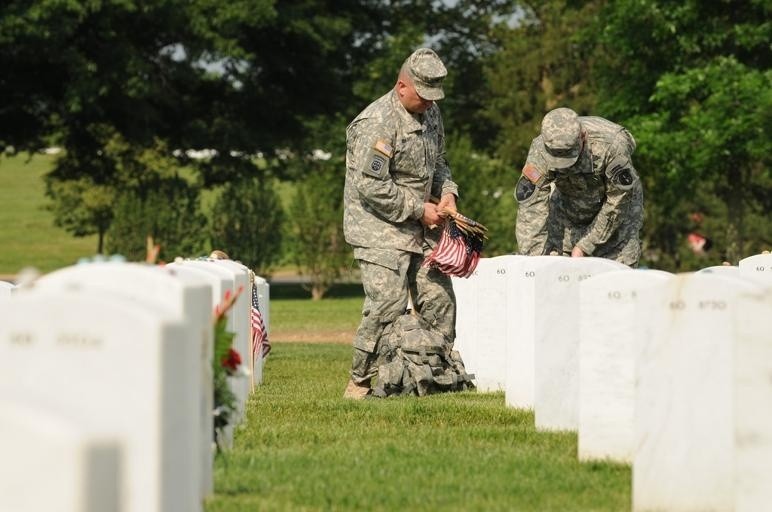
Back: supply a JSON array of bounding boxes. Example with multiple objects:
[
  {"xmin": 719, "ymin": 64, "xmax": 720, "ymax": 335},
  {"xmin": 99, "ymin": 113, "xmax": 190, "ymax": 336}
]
[{"xmin": 376, "ymin": 309, "xmax": 475, "ymax": 396}]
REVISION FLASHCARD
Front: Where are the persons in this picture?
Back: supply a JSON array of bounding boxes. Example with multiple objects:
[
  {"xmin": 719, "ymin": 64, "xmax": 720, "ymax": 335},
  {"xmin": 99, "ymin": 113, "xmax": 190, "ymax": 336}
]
[
  {"xmin": 513, "ymin": 107, "xmax": 645, "ymax": 269},
  {"xmin": 342, "ymin": 48, "xmax": 459, "ymax": 400}
]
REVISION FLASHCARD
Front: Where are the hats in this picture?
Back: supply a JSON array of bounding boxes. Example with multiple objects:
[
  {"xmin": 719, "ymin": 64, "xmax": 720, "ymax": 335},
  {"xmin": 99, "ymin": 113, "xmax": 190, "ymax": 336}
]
[
  {"xmin": 541, "ymin": 107, "xmax": 582, "ymax": 169},
  {"xmin": 406, "ymin": 48, "xmax": 448, "ymax": 101}
]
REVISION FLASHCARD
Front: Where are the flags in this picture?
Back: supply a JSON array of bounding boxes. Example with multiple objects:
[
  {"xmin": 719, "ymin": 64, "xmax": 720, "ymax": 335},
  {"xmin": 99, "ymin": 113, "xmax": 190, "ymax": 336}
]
[
  {"xmin": 420, "ymin": 213, "xmax": 483, "ymax": 279},
  {"xmin": 251, "ymin": 284, "xmax": 271, "ymax": 358}
]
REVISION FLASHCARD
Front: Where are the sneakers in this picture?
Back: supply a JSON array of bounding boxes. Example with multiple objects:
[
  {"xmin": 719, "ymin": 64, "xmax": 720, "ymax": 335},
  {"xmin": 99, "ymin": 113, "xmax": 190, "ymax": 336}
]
[{"xmin": 345, "ymin": 380, "xmax": 372, "ymax": 396}]
